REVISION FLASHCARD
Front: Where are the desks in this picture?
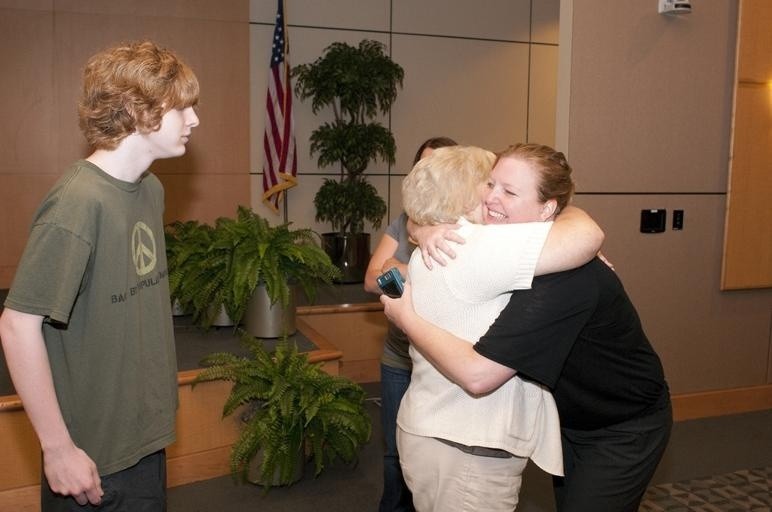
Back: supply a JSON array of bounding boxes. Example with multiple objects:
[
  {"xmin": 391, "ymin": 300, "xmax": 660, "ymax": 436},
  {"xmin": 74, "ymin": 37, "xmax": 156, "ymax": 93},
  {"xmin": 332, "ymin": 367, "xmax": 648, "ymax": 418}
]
[{"xmin": 295, "ymin": 267, "xmax": 386, "ymax": 384}]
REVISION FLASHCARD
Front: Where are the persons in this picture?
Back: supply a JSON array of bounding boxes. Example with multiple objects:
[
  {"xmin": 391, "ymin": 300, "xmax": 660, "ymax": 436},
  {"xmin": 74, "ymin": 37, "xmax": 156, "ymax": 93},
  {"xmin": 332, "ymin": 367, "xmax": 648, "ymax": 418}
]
[
  {"xmin": 364, "ymin": 135, "xmax": 457, "ymax": 512},
  {"xmin": 394, "ymin": 145, "xmax": 606, "ymax": 511},
  {"xmin": 377, "ymin": 143, "xmax": 673, "ymax": 511},
  {"xmin": 1, "ymin": 39, "xmax": 200, "ymax": 512}
]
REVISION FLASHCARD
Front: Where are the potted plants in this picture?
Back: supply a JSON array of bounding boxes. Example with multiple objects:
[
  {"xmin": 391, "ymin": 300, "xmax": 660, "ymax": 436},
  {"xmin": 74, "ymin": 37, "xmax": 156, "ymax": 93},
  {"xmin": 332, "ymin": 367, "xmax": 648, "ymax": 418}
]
[
  {"xmin": 190, "ymin": 322, "xmax": 371, "ymax": 491},
  {"xmin": 165, "ymin": 203, "xmax": 336, "ymax": 338},
  {"xmin": 286, "ymin": 40, "xmax": 405, "ymax": 286}
]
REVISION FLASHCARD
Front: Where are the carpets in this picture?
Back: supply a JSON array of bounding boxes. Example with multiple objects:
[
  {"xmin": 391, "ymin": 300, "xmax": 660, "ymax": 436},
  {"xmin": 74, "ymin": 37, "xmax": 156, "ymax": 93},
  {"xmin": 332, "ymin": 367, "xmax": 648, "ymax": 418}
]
[{"xmin": 637, "ymin": 464, "xmax": 770, "ymax": 510}]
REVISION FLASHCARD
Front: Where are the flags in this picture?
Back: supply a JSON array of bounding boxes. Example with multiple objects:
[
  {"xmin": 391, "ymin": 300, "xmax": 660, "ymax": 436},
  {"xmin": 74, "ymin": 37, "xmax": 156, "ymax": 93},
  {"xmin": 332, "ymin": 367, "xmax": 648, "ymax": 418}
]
[{"xmin": 262, "ymin": 0, "xmax": 298, "ymax": 214}]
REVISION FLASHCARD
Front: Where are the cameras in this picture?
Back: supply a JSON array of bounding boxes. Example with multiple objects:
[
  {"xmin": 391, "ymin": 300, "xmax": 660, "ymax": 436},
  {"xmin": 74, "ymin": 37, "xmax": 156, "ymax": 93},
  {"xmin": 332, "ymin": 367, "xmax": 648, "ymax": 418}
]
[{"xmin": 376, "ymin": 267, "xmax": 405, "ymax": 300}]
